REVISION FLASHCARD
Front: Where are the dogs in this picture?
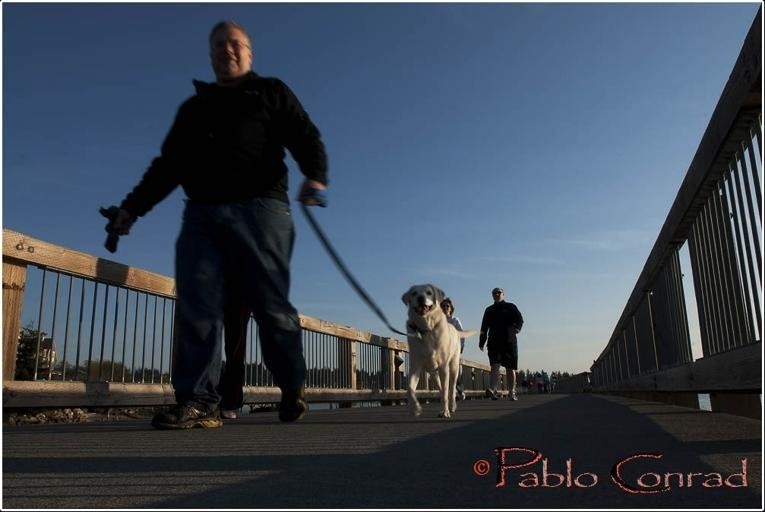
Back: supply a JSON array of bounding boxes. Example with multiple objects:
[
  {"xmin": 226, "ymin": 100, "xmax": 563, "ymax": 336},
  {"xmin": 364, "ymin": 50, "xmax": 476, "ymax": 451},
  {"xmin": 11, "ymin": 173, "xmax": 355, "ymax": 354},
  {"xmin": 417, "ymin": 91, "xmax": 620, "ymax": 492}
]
[{"xmin": 400, "ymin": 283, "xmax": 478, "ymax": 419}]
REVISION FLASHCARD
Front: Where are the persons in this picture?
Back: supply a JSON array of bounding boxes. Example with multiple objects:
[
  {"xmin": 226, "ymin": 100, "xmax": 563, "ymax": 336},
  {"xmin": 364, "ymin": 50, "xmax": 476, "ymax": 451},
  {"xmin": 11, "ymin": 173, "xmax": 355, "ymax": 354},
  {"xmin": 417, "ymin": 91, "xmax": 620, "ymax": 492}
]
[
  {"xmin": 100, "ymin": 19, "xmax": 329, "ymax": 435},
  {"xmin": 478, "ymin": 286, "xmax": 524, "ymax": 400},
  {"xmin": 440, "ymin": 296, "xmax": 467, "ymax": 401}
]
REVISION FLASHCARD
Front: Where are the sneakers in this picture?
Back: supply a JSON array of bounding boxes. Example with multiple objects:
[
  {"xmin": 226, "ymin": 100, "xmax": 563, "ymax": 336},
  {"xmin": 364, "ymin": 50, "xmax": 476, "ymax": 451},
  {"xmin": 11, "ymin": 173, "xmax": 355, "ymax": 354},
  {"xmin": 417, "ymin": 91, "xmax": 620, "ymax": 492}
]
[
  {"xmin": 276, "ymin": 377, "xmax": 307, "ymax": 425},
  {"xmin": 151, "ymin": 400, "xmax": 224, "ymax": 430},
  {"xmin": 456, "ymin": 388, "xmax": 519, "ymax": 403},
  {"xmin": 220, "ymin": 407, "xmax": 237, "ymax": 420}
]
